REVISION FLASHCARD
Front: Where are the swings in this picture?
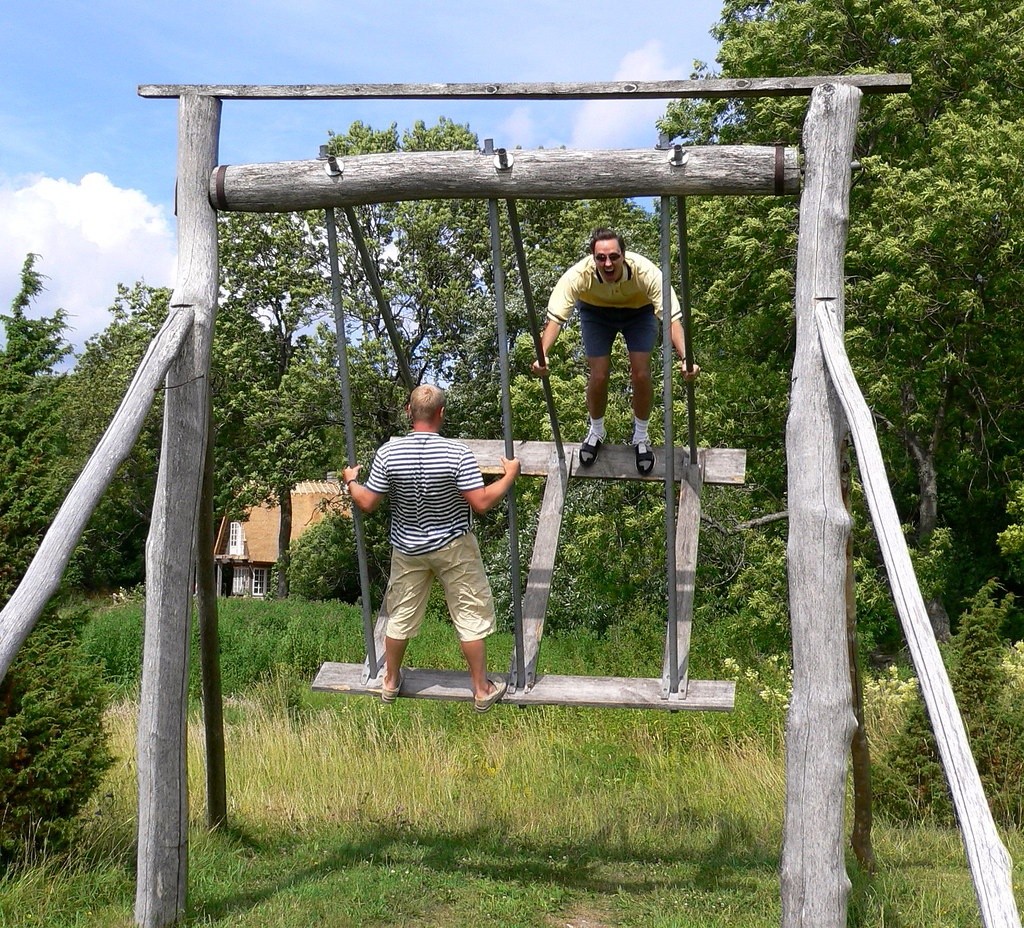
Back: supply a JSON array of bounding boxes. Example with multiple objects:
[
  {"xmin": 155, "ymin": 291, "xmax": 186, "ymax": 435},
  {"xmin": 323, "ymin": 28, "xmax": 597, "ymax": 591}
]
[{"xmin": 308, "ymin": 195, "xmax": 748, "ymax": 711}]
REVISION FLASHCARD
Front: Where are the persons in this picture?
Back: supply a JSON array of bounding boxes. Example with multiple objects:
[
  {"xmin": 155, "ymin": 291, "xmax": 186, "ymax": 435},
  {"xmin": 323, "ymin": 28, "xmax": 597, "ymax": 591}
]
[
  {"xmin": 343, "ymin": 383, "xmax": 521, "ymax": 713},
  {"xmin": 533, "ymin": 226, "xmax": 701, "ymax": 475}
]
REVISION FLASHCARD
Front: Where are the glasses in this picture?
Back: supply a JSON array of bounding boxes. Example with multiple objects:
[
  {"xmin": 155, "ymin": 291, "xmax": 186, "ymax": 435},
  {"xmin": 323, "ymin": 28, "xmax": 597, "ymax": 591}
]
[{"xmin": 595, "ymin": 252, "xmax": 622, "ymax": 262}]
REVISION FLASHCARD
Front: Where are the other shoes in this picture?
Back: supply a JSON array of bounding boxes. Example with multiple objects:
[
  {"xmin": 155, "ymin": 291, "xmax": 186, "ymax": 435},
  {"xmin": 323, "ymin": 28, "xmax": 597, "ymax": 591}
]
[
  {"xmin": 381, "ymin": 668, "xmax": 405, "ymax": 703},
  {"xmin": 474, "ymin": 672, "xmax": 506, "ymax": 713},
  {"xmin": 578, "ymin": 427, "xmax": 608, "ymax": 466},
  {"xmin": 634, "ymin": 433, "xmax": 657, "ymax": 475}
]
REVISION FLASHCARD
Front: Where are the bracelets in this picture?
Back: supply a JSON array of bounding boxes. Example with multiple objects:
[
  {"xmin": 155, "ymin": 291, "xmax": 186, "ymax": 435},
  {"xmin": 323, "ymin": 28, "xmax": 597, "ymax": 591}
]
[
  {"xmin": 682, "ymin": 358, "xmax": 686, "ymax": 364},
  {"xmin": 347, "ymin": 479, "xmax": 357, "ymax": 486}
]
